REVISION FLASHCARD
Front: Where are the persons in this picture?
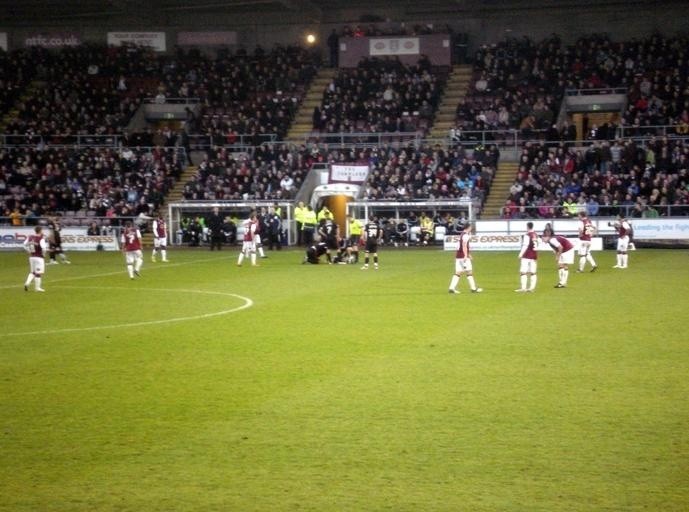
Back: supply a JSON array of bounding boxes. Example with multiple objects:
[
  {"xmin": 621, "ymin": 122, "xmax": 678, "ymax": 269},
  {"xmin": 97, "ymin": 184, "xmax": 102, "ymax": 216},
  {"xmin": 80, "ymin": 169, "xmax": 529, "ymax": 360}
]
[{"xmin": 0, "ymin": 14, "xmax": 688, "ymax": 294}]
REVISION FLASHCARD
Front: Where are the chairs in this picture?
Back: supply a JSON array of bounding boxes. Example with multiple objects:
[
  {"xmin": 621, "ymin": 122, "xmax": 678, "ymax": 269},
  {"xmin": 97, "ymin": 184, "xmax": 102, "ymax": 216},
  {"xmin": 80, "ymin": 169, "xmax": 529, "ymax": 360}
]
[{"xmin": 0, "ymin": 50, "xmax": 688, "ymax": 226}]
